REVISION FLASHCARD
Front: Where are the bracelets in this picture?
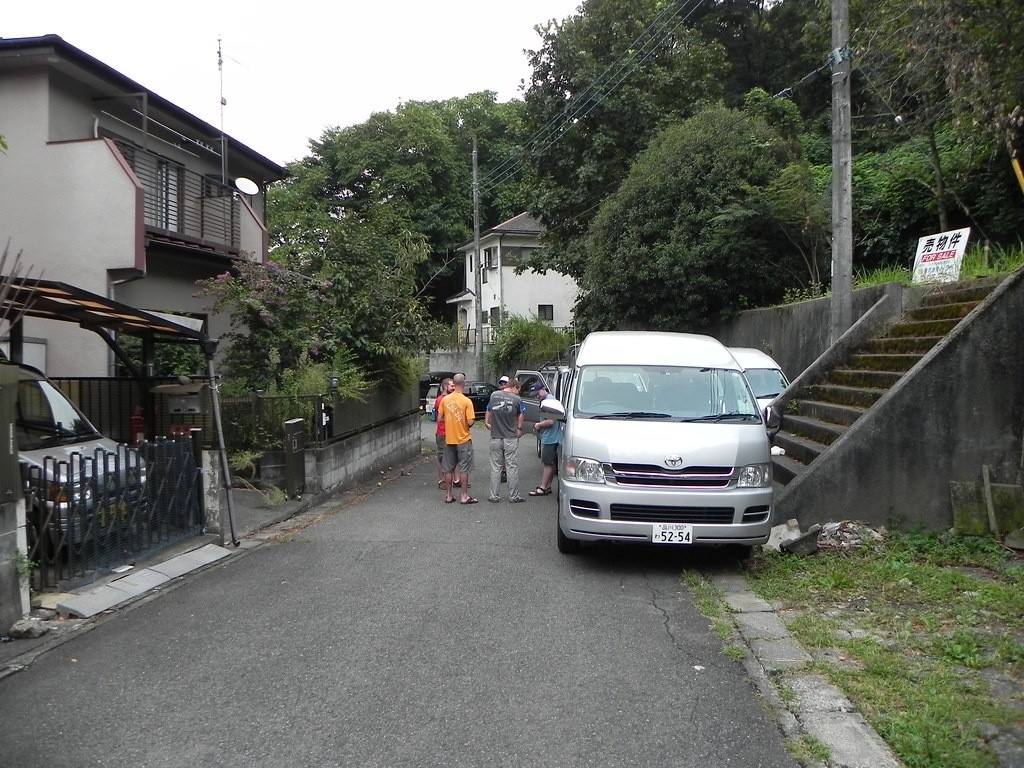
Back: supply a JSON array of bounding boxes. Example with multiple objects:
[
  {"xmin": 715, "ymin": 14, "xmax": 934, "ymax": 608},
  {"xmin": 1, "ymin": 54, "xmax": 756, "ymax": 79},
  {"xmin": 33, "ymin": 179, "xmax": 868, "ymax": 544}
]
[{"xmin": 518, "ymin": 427, "xmax": 522, "ymax": 430}]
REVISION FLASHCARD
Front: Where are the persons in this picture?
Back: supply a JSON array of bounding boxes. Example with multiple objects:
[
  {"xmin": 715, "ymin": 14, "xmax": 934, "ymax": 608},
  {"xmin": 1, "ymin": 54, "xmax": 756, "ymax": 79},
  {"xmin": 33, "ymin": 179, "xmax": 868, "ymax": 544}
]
[
  {"xmin": 528, "ymin": 382, "xmax": 561, "ymax": 495},
  {"xmin": 485, "ymin": 376, "xmax": 526, "ymax": 503},
  {"xmin": 436, "ymin": 374, "xmax": 479, "ymax": 504}
]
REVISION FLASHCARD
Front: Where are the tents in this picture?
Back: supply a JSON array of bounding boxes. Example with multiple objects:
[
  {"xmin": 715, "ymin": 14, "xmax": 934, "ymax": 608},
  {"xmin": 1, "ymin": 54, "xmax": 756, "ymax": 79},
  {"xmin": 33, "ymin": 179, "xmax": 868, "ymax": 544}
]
[{"xmin": 0, "ymin": 276, "xmax": 241, "ymax": 547}]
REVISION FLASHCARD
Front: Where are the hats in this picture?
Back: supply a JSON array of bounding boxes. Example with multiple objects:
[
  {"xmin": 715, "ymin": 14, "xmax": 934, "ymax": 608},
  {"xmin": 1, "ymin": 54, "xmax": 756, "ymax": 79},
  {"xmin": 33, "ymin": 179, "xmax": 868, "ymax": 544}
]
[
  {"xmin": 499, "ymin": 376, "xmax": 510, "ymax": 384},
  {"xmin": 528, "ymin": 382, "xmax": 544, "ymax": 396}
]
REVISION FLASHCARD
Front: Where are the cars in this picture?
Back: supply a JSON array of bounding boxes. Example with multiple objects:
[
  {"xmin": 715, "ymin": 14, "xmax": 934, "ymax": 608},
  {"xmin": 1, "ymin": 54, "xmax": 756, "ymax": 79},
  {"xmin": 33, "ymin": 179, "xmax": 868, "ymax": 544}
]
[{"xmin": 419, "ymin": 364, "xmax": 650, "ymax": 421}]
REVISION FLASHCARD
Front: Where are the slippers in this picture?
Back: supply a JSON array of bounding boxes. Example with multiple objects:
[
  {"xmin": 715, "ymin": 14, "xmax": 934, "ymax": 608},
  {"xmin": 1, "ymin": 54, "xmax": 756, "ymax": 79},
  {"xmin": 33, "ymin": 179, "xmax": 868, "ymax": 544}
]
[
  {"xmin": 460, "ymin": 496, "xmax": 478, "ymax": 504},
  {"xmin": 445, "ymin": 495, "xmax": 456, "ymax": 503}
]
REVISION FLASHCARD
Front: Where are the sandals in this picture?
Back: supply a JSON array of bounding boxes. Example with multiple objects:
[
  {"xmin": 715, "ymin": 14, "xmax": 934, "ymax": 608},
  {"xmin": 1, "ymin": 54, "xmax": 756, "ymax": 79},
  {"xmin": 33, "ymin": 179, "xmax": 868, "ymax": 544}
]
[{"xmin": 528, "ymin": 486, "xmax": 553, "ymax": 496}]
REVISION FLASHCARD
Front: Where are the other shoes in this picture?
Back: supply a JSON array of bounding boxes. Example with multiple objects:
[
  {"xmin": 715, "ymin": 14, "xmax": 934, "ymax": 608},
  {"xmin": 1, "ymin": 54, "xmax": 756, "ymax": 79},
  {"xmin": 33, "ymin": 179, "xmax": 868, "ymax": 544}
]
[
  {"xmin": 488, "ymin": 497, "xmax": 499, "ymax": 503},
  {"xmin": 500, "ymin": 471, "xmax": 507, "ymax": 483},
  {"xmin": 439, "ymin": 480, "xmax": 446, "ymax": 490},
  {"xmin": 452, "ymin": 480, "xmax": 471, "ymax": 488},
  {"xmin": 509, "ymin": 498, "xmax": 526, "ymax": 502}
]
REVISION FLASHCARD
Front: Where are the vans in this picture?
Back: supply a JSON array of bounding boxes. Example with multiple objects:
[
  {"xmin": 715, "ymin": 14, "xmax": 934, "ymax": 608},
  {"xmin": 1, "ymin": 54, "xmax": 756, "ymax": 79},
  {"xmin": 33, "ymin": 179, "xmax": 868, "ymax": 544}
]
[
  {"xmin": 539, "ymin": 330, "xmax": 783, "ymax": 554},
  {"xmin": 0, "ymin": 347, "xmax": 148, "ymax": 568},
  {"xmin": 713, "ymin": 347, "xmax": 791, "ymax": 419}
]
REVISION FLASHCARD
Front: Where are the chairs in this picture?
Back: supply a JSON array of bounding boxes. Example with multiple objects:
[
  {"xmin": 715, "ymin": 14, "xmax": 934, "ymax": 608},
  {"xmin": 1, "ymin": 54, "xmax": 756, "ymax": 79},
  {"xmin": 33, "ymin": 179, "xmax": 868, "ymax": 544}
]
[
  {"xmin": 589, "ymin": 377, "xmax": 614, "ymax": 405},
  {"xmin": 647, "ymin": 377, "xmax": 674, "ymax": 407},
  {"xmin": 750, "ymin": 375, "xmax": 769, "ymax": 396}
]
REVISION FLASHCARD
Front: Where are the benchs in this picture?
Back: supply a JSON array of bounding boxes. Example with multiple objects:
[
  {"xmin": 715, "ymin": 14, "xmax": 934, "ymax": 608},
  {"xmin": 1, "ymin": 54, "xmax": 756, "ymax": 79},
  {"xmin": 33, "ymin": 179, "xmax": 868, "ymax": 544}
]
[{"xmin": 582, "ymin": 381, "xmax": 639, "ymax": 411}]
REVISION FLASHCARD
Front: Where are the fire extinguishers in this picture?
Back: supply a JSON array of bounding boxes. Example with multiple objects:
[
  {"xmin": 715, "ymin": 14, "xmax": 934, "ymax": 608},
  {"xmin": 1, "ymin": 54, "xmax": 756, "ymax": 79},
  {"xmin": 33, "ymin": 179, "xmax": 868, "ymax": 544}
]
[{"xmin": 129, "ymin": 406, "xmax": 145, "ymax": 445}]
